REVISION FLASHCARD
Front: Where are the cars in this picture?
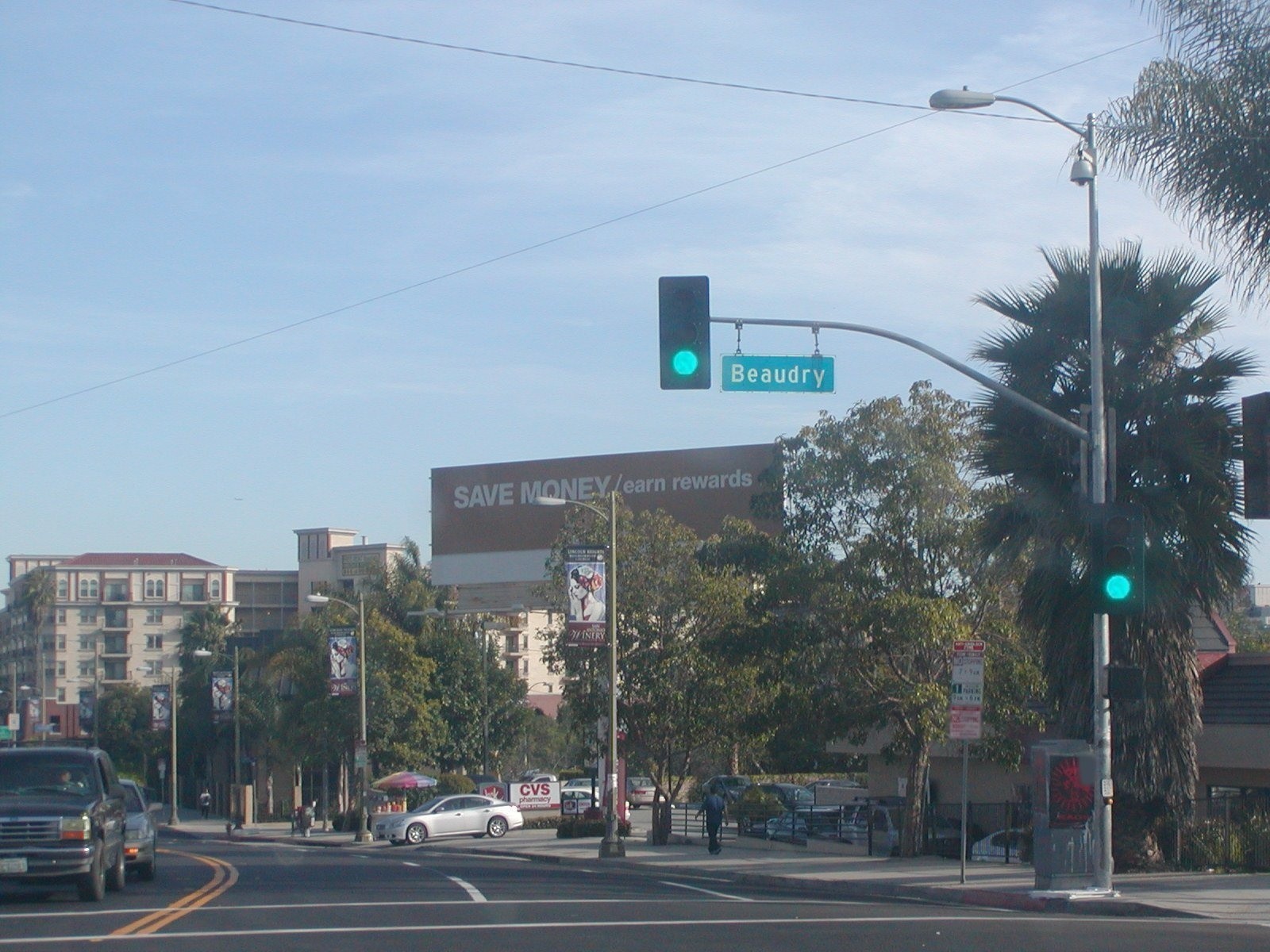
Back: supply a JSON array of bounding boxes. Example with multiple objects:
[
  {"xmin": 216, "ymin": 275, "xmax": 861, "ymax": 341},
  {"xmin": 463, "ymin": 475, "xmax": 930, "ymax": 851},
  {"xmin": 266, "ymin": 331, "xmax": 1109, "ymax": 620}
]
[
  {"xmin": 966, "ymin": 827, "xmax": 1026, "ymax": 862},
  {"xmin": 117, "ymin": 778, "xmax": 165, "ymax": 879},
  {"xmin": 697, "ymin": 774, "xmax": 971, "ymax": 857},
  {"xmin": 625, "ymin": 776, "xmax": 672, "ymax": 809},
  {"xmin": 377, "ymin": 793, "xmax": 525, "ymax": 845},
  {"xmin": 523, "ymin": 773, "xmax": 631, "ymax": 821}
]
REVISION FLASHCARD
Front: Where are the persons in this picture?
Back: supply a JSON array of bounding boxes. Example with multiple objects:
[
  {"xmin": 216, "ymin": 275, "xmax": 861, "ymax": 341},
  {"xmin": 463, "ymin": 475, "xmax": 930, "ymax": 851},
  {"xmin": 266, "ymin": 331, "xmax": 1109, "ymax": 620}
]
[
  {"xmin": 56, "ymin": 770, "xmax": 84, "ymax": 787},
  {"xmin": 568, "ymin": 565, "xmax": 605, "ymax": 622},
  {"xmin": 199, "ymin": 788, "xmax": 211, "ymax": 820},
  {"xmin": 695, "ymin": 785, "xmax": 729, "ymax": 854}
]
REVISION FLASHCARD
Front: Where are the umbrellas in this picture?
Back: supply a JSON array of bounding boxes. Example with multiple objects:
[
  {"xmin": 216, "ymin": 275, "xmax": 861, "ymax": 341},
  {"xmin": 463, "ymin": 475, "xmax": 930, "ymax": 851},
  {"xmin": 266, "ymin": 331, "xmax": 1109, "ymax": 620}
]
[{"xmin": 372, "ymin": 771, "xmax": 438, "ymax": 788}]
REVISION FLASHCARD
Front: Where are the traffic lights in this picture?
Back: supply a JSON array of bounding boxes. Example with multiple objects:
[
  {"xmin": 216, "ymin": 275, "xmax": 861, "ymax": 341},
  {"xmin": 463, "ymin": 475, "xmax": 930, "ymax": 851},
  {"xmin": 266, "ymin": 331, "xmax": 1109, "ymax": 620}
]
[
  {"xmin": 1086, "ymin": 500, "xmax": 1147, "ymax": 618},
  {"xmin": 656, "ymin": 273, "xmax": 712, "ymax": 389}
]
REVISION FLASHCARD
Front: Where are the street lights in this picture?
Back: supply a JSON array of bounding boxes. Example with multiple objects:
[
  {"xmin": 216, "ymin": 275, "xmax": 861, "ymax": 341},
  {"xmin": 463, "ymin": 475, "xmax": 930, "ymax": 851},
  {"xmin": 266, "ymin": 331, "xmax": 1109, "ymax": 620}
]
[
  {"xmin": 134, "ymin": 665, "xmax": 183, "ymax": 827},
  {"xmin": 527, "ymin": 492, "xmax": 626, "ymax": 860},
  {"xmin": 304, "ymin": 593, "xmax": 373, "ymax": 843},
  {"xmin": 16, "ymin": 683, "xmax": 49, "ymax": 743},
  {"xmin": 926, "ymin": 89, "xmax": 1119, "ymax": 898},
  {"xmin": 192, "ymin": 644, "xmax": 245, "ymax": 833}
]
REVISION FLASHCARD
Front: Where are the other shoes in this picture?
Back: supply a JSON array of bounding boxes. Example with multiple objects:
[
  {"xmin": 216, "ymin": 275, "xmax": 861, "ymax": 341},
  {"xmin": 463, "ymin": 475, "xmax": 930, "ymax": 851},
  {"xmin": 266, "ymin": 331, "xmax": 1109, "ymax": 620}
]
[{"xmin": 715, "ymin": 848, "xmax": 721, "ymax": 855}]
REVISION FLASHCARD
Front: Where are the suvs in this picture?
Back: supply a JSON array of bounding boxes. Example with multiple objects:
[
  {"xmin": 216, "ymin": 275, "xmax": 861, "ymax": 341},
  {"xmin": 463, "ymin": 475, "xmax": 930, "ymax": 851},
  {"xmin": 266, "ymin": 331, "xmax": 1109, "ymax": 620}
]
[{"xmin": 0, "ymin": 743, "xmax": 128, "ymax": 902}]
